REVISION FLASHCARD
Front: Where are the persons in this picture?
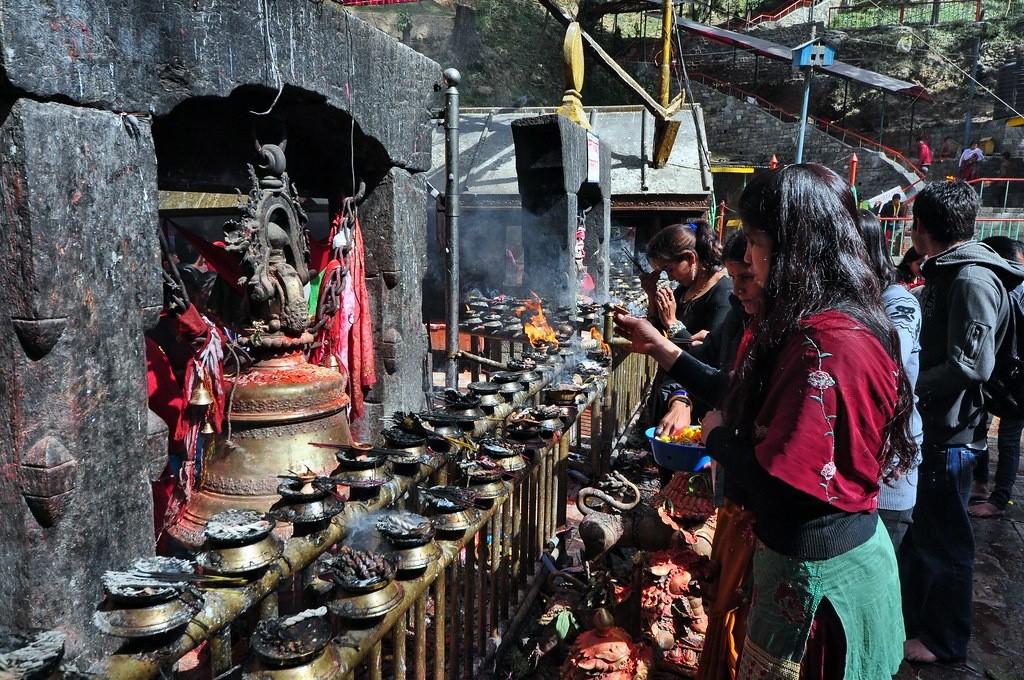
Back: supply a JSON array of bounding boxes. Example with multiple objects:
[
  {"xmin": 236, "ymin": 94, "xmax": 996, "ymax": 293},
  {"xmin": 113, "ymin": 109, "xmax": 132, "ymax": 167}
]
[
  {"xmin": 915, "ymin": 136, "xmax": 932, "ymax": 174},
  {"xmin": 611, "ymin": 164, "xmax": 919, "ymax": 680},
  {"xmin": 958, "ymin": 141, "xmax": 984, "ymax": 181},
  {"xmin": 638, "ymin": 180, "xmax": 1024, "ymax": 661},
  {"xmin": 998, "ymin": 151, "xmax": 1015, "ymax": 178}
]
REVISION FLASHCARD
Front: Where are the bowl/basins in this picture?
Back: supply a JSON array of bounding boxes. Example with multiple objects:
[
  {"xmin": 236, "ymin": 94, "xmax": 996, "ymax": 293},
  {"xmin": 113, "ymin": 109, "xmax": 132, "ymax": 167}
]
[{"xmin": 645, "ymin": 425, "xmax": 712, "ymax": 472}]
